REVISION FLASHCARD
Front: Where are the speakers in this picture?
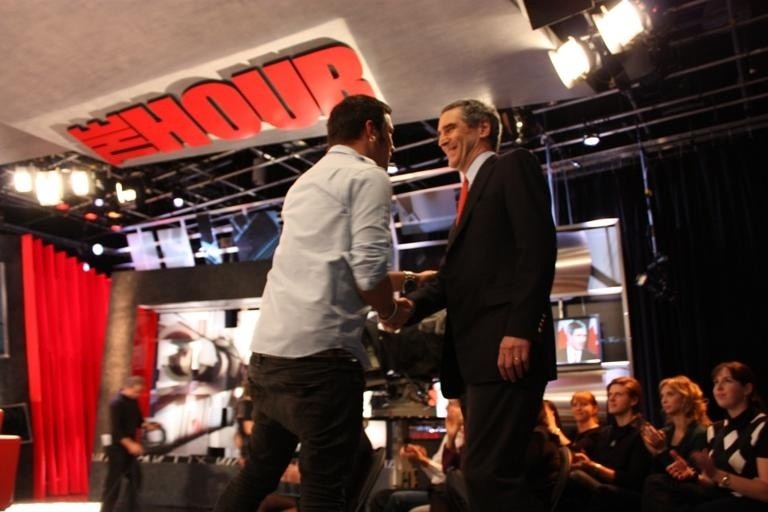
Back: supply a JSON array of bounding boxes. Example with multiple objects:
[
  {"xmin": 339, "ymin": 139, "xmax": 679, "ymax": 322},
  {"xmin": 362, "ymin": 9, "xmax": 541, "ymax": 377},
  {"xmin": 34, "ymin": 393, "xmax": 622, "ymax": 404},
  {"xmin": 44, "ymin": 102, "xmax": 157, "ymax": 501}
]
[{"xmin": 233, "ymin": 210, "xmax": 282, "ymax": 263}]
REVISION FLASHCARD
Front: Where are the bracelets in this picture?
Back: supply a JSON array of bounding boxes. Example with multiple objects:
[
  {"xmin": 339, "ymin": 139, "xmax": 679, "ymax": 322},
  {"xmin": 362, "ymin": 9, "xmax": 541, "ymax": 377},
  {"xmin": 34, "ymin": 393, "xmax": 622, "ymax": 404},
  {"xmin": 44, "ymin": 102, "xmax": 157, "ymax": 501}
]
[{"xmin": 378, "ymin": 301, "xmax": 399, "ymax": 324}]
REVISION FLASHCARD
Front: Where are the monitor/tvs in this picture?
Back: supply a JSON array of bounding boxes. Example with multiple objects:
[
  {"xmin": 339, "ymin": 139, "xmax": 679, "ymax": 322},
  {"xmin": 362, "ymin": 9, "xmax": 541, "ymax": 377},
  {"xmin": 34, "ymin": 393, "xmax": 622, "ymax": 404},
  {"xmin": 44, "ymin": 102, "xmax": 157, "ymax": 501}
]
[{"xmin": 553, "ymin": 314, "xmax": 606, "ymax": 367}]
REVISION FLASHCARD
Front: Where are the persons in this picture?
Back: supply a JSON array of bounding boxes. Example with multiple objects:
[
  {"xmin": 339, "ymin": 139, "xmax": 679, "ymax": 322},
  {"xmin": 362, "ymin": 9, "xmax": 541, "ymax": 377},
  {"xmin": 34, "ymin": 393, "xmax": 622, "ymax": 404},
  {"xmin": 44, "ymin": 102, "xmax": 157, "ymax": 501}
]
[
  {"xmin": 231, "ymin": 390, "xmax": 300, "ymax": 481},
  {"xmin": 546, "ymin": 361, "xmax": 768, "ymax": 511},
  {"xmin": 216, "ymin": 93, "xmax": 439, "ymax": 511},
  {"xmin": 403, "ymin": 94, "xmax": 557, "ymax": 510},
  {"xmin": 101, "ymin": 376, "xmax": 161, "ymax": 511},
  {"xmin": 370, "ymin": 403, "xmax": 469, "ymax": 511},
  {"xmin": 556, "ymin": 320, "xmax": 595, "ymax": 364}
]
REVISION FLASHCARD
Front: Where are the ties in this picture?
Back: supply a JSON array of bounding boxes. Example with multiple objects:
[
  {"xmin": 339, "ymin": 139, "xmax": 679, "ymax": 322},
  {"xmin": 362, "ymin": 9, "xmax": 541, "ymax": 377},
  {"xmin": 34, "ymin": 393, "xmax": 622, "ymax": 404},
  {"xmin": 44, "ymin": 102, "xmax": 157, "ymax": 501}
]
[{"xmin": 455, "ymin": 176, "xmax": 469, "ymax": 225}]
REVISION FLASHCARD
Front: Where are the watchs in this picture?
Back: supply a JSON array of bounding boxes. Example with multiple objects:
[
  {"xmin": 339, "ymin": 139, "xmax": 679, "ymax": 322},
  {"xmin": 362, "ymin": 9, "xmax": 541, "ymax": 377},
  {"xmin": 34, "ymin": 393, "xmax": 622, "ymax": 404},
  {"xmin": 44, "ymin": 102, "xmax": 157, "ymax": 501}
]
[{"xmin": 402, "ymin": 271, "xmax": 417, "ymax": 296}]
